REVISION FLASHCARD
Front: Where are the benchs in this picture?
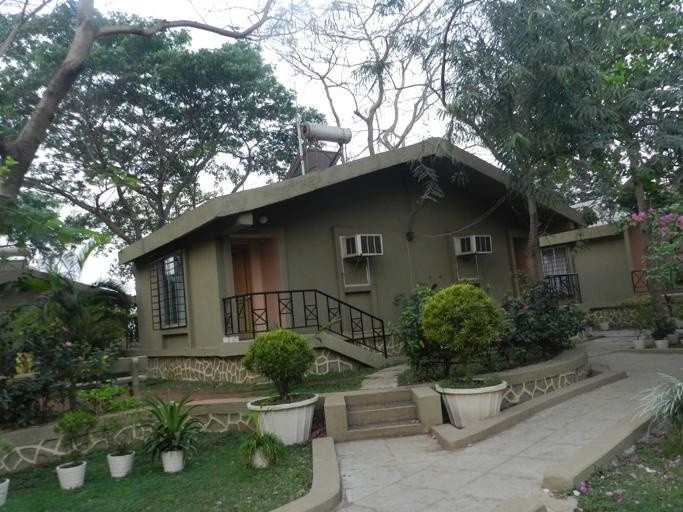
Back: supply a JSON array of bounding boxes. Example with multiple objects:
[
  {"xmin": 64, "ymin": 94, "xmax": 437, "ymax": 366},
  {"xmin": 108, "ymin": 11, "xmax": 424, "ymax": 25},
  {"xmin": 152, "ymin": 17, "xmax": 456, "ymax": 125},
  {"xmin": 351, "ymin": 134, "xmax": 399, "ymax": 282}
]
[{"xmin": 52, "ymin": 353, "xmax": 148, "ymax": 407}]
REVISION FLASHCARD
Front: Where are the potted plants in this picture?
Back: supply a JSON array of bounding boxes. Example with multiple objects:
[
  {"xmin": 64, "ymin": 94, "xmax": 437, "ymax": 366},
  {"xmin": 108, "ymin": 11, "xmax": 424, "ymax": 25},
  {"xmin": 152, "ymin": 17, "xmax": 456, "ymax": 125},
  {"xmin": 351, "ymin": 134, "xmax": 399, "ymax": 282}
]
[
  {"xmin": 239, "ymin": 327, "xmax": 317, "ymax": 445},
  {"xmin": 131, "ymin": 390, "xmax": 193, "ymax": 471},
  {"xmin": 651, "ymin": 322, "xmax": 671, "ymax": 350},
  {"xmin": 423, "ymin": 281, "xmax": 509, "ymax": 428},
  {"xmin": 229, "ymin": 419, "xmax": 279, "ymax": 470},
  {"xmin": 81, "ymin": 384, "xmax": 144, "ymax": 479},
  {"xmin": 50, "ymin": 408, "xmax": 96, "ymax": 491},
  {"xmin": 617, "ymin": 296, "xmax": 656, "ymax": 349},
  {"xmin": 0, "ymin": 436, "xmax": 15, "ymax": 507},
  {"xmin": 666, "ymin": 323, "xmax": 680, "ymax": 345}
]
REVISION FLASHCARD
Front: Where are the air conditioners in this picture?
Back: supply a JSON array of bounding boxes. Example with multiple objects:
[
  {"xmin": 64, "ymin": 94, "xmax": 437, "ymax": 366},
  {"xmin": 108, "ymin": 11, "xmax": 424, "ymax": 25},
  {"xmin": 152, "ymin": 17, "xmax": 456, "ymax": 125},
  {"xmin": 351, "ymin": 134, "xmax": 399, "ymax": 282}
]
[
  {"xmin": 455, "ymin": 233, "xmax": 492, "ymax": 257},
  {"xmin": 342, "ymin": 231, "xmax": 383, "ymax": 260}
]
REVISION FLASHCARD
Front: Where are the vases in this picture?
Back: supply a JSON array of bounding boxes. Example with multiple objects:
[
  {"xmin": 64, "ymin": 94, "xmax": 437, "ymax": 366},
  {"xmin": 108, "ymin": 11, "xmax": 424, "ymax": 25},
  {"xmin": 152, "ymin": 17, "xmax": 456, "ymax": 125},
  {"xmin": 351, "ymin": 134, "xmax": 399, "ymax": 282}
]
[{"xmin": 598, "ymin": 322, "xmax": 609, "ymax": 331}]
